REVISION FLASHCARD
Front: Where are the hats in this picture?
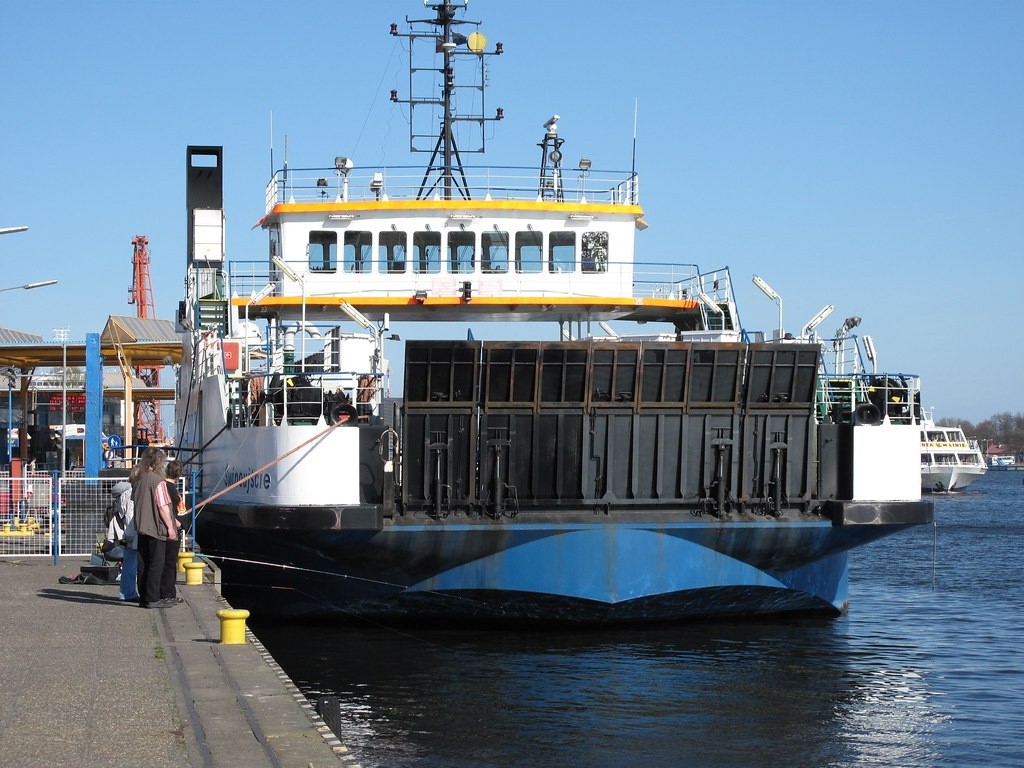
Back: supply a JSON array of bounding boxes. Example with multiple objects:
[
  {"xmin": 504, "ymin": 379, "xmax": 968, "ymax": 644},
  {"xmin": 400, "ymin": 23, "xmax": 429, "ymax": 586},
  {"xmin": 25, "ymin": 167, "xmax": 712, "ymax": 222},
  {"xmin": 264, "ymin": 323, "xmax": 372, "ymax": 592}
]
[{"xmin": 111, "ymin": 481, "xmax": 131, "ymax": 498}]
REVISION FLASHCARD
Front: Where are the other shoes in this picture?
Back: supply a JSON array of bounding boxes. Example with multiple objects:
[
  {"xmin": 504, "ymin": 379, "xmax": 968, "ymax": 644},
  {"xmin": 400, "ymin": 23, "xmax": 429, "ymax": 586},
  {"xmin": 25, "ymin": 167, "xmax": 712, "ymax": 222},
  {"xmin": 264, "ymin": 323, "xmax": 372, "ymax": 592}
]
[{"xmin": 128, "ymin": 598, "xmax": 141, "ymax": 602}]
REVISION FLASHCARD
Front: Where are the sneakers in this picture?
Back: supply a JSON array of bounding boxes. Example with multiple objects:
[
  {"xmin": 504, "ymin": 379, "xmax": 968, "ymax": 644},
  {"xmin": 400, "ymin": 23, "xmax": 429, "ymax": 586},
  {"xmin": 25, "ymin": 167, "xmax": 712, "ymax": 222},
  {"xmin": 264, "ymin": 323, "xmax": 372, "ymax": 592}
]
[
  {"xmin": 139, "ymin": 600, "xmax": 175, "ymax": 608},
  {"xmin": 161, "ymin": 597, "xmax": 184, "ymax": 604}
]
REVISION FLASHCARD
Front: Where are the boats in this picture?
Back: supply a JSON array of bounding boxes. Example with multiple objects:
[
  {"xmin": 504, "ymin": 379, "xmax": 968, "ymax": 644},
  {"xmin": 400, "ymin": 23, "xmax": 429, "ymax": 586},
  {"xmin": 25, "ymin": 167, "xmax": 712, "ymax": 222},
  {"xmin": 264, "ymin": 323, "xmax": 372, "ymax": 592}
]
[
  {"xmin": 170, "ymin": 2, "xmax": 937, "ymax": 620},
  {"xmin": 917, "ymin": 422, "xmax": 986, "ymax": 493}
]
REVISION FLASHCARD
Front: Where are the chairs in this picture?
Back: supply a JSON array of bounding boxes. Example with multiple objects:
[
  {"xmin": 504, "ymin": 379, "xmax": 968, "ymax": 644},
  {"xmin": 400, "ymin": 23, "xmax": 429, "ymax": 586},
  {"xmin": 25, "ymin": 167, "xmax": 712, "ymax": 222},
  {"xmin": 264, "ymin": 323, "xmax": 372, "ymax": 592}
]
[{"xmin": 95, "ymin": 530, "xmax": 121, "ymax": 568}]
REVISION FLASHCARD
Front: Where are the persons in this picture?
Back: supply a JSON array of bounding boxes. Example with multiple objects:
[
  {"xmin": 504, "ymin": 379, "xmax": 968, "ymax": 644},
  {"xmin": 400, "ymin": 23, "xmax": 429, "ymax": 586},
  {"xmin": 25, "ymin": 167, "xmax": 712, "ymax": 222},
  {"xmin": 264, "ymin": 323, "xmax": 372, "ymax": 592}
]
[
  {"xmin": 130, "ymin": 448, "xmax": 177, "ymax": 608},
  {"xmin": 162, "ymin": 460, "xmax": 186, "ymax": 603},
  {"xmin": 102, "ymin": 466, "xmax": 141, "ymax": 602}
]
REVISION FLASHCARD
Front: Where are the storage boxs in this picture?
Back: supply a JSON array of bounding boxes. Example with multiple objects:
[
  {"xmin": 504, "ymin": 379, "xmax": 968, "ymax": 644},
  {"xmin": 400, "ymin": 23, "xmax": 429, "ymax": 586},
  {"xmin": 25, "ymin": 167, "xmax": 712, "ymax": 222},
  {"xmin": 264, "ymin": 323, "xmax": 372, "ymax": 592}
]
[{"xmin": 80, "ymin": 565, "xmax": 119, "ymax": 581}]
